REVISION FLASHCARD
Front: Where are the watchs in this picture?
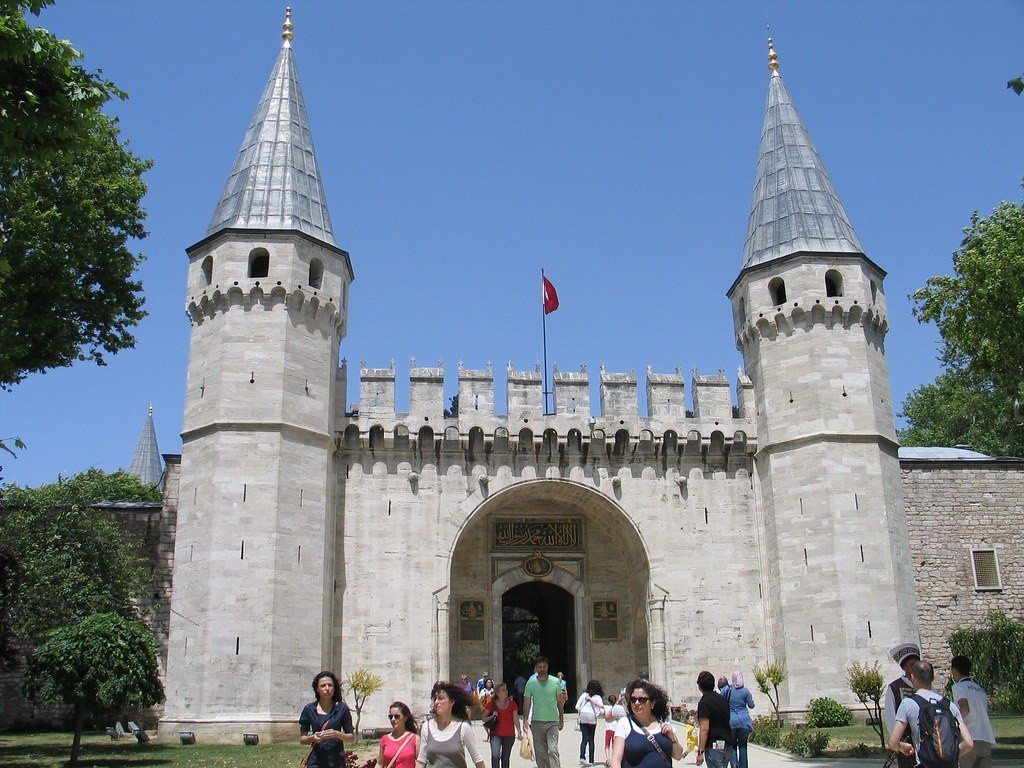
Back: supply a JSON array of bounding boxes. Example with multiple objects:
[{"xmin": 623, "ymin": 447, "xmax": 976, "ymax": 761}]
[{"xmin": 672, "ymin": 737, "xmax": 677, "ymax": 744}]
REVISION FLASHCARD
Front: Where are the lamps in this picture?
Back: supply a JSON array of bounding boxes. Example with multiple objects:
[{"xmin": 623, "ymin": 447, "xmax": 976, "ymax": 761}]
[
  {"xmin": 105, "ymin": 721, "xmax": 150, "ymax": 746},
  {"xmin": 361, "ymin": 728, "xmax": 376, "ymax": 740},
  {"xmin": 180, "ymin": 731, "xmax": 195, "ymax": 746},
  {"xmin": 865, "ymin": 717, "xmax": 881, "ymax": 725},
  {"xmin": 242, "ymin": 733, "xmax": 259, "ymax": 746},
  {"xmin": 795, "ymin": 722, "xmax": 811, "ymax": 728}
]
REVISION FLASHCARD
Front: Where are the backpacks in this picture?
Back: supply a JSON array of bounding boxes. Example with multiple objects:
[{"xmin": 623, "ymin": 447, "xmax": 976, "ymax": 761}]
[{"xmin": 907, "ymin": 694, "xmax": 960, "ymax": 768}]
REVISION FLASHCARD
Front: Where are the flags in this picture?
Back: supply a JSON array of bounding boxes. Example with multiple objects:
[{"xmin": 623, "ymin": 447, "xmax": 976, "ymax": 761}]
[{"xmin": 543, "ymin": 275, "xmax": 559, "ymax": 315}]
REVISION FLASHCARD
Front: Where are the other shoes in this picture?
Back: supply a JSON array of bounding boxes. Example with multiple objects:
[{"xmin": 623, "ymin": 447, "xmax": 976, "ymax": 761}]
[
  {"xmin": 589, "ymin": 762, "xmax": 596, "ymax": 766},
  {"xmin": 580, "ymin": 759, "xmax": 591, "ymax": 766}
]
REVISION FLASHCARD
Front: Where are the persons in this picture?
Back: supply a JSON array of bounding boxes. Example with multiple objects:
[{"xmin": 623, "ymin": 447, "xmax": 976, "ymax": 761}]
[
  {"xmin": 682, "ymin": 670, "xmax": 755, "ymax": 768},
  {"xmin": 482, "ymin": 683, "xmax": 523, "ymax": 768},
  {"xmin": 610, "ymin": 680, "xmax": 683, "ymax": 768},
  {"xmin": 416, "ymin": 681, "xmax": 486, "ymax": 768},
  {"xmin": 522, "ymin": 655, "xmax": 565, "ymax": 768},
  {"xmin": 453, "ymin": 672, "xmax": 494, "ymax": 725},
  {"xmin": 575, "ymin": 680, "xmax": 628, "ymax": 767},
  {"xmin": 298, "ymin": 671, "xmax": 355, "ymax": 768},
  {"xmin": 884, "ymin": 642, "xmax": 996, "ymax": 768},
  {"xmin": 374, "ymin": 701, "xmax": 421, "ymax": 768},
  {"xmin": 514, "ymin": 672, "xmax": 569, "ymax": 714}
]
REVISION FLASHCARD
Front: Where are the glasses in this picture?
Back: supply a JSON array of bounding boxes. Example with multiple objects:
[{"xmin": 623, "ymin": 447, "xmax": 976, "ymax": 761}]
[
  {"xmin": 630, "ymin": 696, "xmax": 651, "ymax": 703},
  {"xmin": 388, "ymin": 715, "xmax": 400, "ymax": 719}
]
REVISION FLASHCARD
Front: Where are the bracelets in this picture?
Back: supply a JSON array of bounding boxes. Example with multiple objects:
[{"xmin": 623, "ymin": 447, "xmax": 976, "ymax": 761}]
[{"xmin": 697, "ymin": 750, "xmax": 704, "ymax": 755}]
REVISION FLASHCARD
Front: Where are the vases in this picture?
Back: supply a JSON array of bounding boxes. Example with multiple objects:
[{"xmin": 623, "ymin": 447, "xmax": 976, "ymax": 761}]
[{"xmin": 772, "ymin": 719, "xmax": 784, "ymax": 728}]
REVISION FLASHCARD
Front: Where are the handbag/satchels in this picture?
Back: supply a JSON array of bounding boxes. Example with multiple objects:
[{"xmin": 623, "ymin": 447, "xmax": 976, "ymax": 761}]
[
  {"xmin": 519, "ymin": 731, "xmax": 534, "ymax": 761},
  {"xmin": 299, "ymin": 757, "xmax": 307, "ymax": 768},
  {"xmin": 484, "ymin": 700, "xmax": 498, "ymax": 729},
  {"xmin": 574, "ymin": 719, "xmax": 582, "ymax": 732}
]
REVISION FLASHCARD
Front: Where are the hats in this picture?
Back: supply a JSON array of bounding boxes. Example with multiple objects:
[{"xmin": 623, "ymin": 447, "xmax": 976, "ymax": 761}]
[
  {"xmin": 483, "ymin": 672, "xmax": 488, "ymax": 675},
  {"xmin": 890, "ymin": 643, "xmax": 920, "ymax": 666}
]
